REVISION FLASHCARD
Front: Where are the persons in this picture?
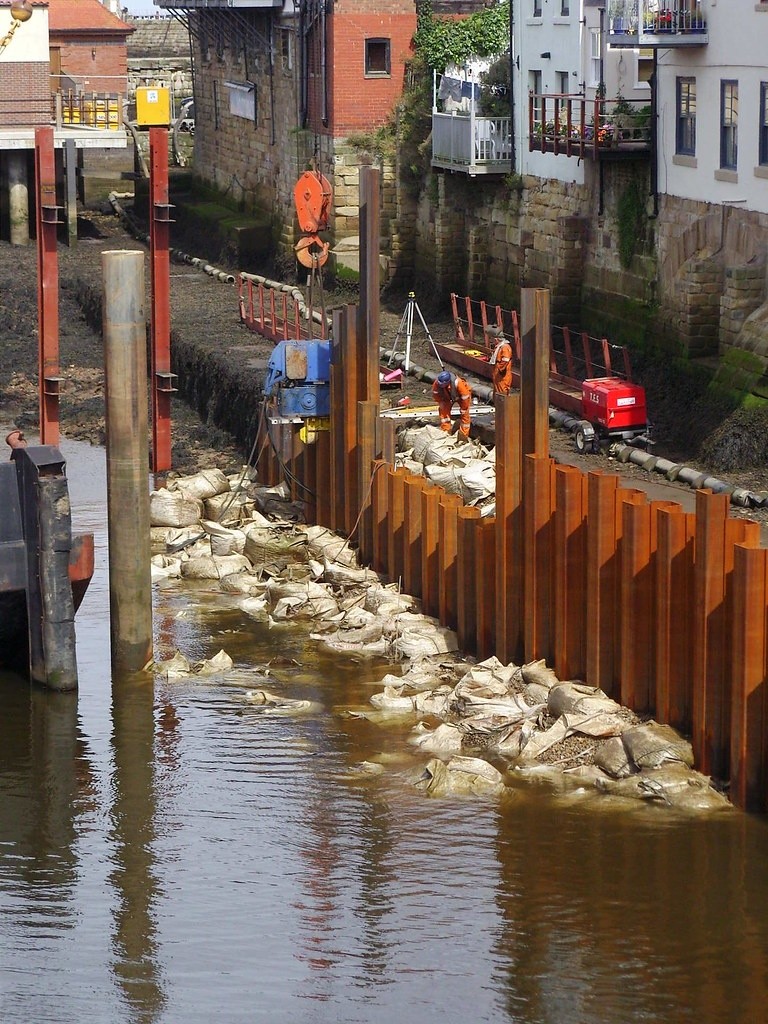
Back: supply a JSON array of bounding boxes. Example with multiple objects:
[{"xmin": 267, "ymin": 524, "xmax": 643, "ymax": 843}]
[
  {"xmin": 489, "ymin": 329, "xmax": 512, "ymax": 405},
  {"xmin": 432, "ymin": 370, "xmax": 472, "ymax": 442}
]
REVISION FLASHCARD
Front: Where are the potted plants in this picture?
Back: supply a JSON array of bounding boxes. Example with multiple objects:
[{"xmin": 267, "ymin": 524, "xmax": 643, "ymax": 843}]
[
  {"xmin": 608, "ymin": 0, "xmax": 657, "ymax": 36},
  {"xmin": 684, "ymin": 10, "xmax": 706, "ymax": 34},
  {"xmin": 643, "ymin": 11, "xmax": 657, "ymax": 34}
]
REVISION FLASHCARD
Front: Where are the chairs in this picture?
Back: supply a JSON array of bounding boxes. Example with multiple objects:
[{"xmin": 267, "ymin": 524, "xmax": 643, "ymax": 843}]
[{"xmin": 475, "ymin": 120, "xmax": 496, "ymax": 159}]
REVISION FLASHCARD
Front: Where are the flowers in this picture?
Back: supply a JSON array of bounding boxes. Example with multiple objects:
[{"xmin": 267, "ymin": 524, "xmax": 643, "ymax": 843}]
[
  {"xmin": 655, "ymin": 9, "xmax": 671, "ymax": 22},
  {"xmin": 585, "ymin": 127, "xmax": 594, "ymax": 140},
  {"xmin": 598, "ymin": 125, "xmax": 623, "ymax": 141}
]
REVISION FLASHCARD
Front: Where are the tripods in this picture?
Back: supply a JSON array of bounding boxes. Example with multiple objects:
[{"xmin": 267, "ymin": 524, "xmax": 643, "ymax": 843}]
[{"xmin": 387, "ymin": 292, "xmax": 445, "ymax": 377}]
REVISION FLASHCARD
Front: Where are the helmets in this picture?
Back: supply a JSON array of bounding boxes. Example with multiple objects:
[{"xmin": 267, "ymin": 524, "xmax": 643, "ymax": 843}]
[
  {"xmin": 489, "ymin": 327, "xmax": 504, "ymax": 339},
  {"xmin": 438, "ymin": 371, "xmax": 451, "ymax": 384}
]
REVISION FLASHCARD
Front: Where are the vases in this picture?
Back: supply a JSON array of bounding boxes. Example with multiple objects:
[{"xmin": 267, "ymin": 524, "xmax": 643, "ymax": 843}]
[{"xmin": 657, "ymin": 21, "xmax": 678, "ymax": 34}]
